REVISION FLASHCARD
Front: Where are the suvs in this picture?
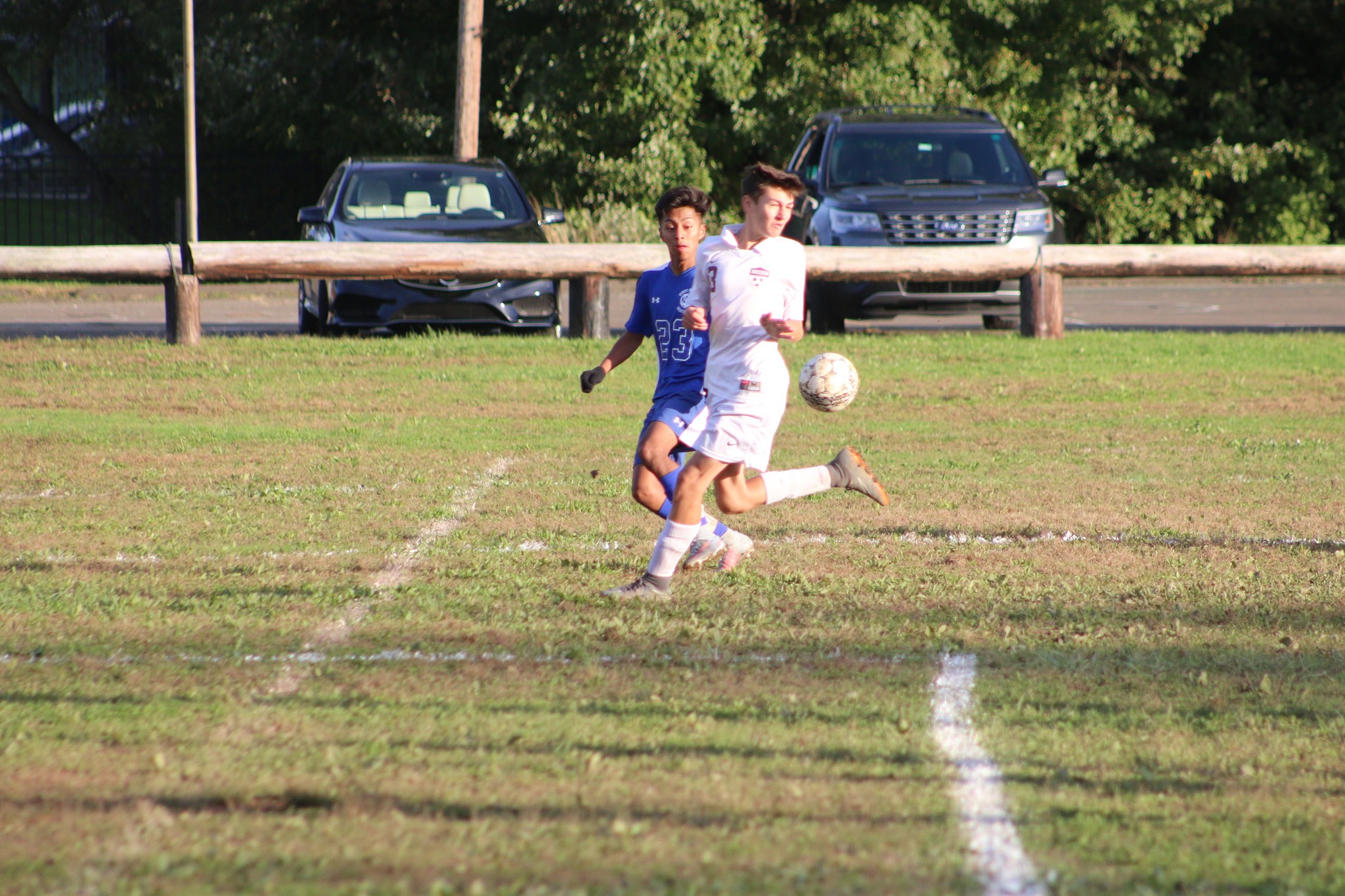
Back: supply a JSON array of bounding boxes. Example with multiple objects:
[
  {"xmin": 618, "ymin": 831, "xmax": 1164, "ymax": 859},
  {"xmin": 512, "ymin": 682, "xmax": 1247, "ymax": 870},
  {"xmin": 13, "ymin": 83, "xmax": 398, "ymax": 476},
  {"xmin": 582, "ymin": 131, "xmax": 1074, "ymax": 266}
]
[{"xmin": 774, "ymin": 108, "xmax": 1069, "ymax": 337}]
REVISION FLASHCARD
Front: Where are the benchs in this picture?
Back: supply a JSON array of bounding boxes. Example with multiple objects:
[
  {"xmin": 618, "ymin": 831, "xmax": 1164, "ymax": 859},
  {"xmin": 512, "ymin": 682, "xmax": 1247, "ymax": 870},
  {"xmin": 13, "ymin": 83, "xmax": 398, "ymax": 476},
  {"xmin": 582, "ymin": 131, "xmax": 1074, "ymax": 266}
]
[{"xmin": 350, "ymin": 186, "xmax": 458, "ymax": 215}]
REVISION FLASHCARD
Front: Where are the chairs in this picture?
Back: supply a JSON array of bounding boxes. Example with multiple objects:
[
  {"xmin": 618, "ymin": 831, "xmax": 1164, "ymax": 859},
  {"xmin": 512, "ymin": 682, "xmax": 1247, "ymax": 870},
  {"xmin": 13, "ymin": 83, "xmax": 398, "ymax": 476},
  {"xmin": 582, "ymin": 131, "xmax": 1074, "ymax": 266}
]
[
  {"xmin": 944, "ymin": 151, "xmax": 975, "ymax": 179},
  {"xmin": 459, "ymin": 184, "xmax": 491, "ymax": 211},
  {"xmin": 357, "ymin": 180, "xmax": 391, "ymax": 218}
]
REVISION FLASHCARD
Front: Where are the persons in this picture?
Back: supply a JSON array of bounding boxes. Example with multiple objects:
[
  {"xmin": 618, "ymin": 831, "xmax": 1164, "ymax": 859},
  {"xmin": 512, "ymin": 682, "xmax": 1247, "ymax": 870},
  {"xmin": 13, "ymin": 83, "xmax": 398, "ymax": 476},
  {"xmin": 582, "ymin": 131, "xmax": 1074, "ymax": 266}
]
[
  {"xmin": 577, "ymin": 187, "xmax": 753, "ymax": 572},
  {"xmin": 622, "ymin": 161, "xmax": 888, "ymax": 596}
]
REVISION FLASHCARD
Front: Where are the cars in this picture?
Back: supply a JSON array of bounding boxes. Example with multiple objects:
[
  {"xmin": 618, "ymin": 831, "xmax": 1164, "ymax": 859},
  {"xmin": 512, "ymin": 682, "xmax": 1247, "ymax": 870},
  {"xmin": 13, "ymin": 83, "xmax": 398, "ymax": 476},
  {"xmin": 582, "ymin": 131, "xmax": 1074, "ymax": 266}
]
[{"xmin": 292, "ymin": 154, "xmax": 568, "ymax": 340}]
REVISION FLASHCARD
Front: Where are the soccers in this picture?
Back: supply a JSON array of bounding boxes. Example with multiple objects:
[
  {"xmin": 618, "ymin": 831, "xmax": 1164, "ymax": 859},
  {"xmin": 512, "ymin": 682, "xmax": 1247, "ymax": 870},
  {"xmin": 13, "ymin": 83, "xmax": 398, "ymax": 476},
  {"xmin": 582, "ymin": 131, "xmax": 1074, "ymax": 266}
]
[{"xmin": 798, "ymin": 353, "xmax": 859, "ymax": 412}]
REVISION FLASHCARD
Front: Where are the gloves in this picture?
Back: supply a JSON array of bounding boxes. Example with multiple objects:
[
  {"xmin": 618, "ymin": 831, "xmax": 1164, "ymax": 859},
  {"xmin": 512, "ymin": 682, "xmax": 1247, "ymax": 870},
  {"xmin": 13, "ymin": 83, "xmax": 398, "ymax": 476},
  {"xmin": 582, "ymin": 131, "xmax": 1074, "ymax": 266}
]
[{"xmin": 580, "ymin": 366, "xmax": 606, "ymax": 393}]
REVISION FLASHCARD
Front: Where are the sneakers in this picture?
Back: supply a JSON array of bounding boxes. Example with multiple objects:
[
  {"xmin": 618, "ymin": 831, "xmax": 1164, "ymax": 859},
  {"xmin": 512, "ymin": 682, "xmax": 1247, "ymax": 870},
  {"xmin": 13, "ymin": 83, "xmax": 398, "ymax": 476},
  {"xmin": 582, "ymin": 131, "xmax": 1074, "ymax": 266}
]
[
  {"xmin": 683, "ymin": 534, "xmax": 726, "ymax": 574},
  {"xmin": 601, "ymin": 577, "xmax": 673, "ymax": 602},
  {"xmin": 712, "ymin": 532, "xmax": 754, "ymax": 574},
  {"xmin": 825, "ymin": 446, "xmax": 889, "ymax": 506}
]
